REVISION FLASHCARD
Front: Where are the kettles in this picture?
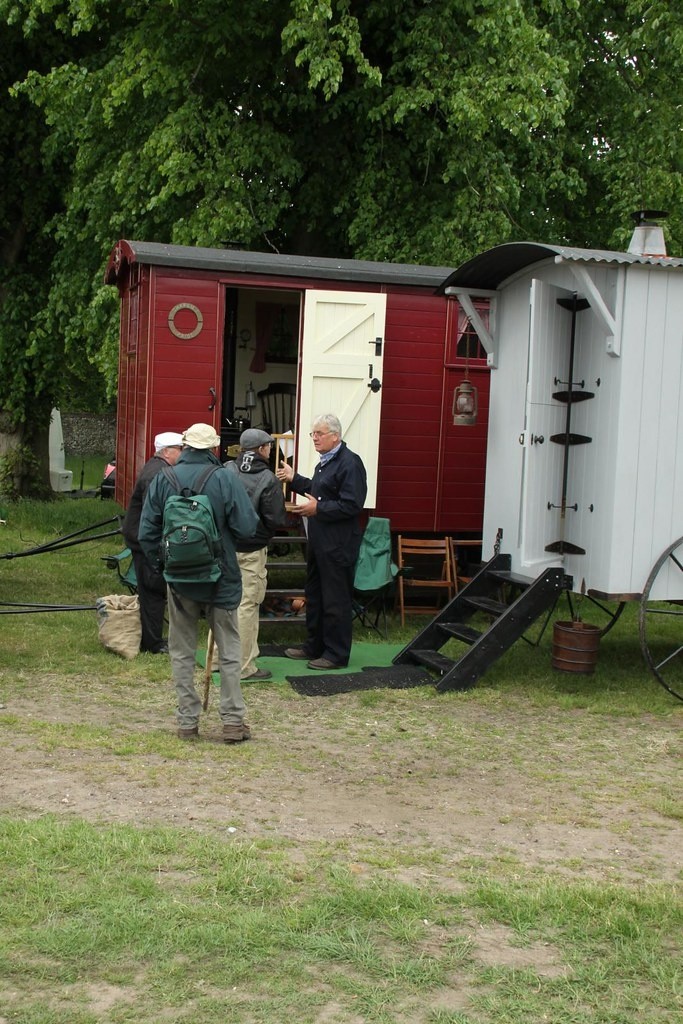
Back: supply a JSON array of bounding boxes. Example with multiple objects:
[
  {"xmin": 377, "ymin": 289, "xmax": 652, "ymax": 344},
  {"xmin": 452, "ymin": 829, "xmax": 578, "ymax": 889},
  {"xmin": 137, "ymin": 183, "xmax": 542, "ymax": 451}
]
[{"xmin": 224, "ymin": 407, "xmax": 252, "ymax": 432}]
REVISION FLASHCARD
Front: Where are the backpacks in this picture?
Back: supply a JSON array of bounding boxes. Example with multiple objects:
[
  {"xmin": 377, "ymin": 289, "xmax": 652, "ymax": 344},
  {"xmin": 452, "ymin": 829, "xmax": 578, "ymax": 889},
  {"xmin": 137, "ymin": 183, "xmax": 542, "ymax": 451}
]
[{"xmin": 158, "ymin": 466, "xmax": 228, "ymax": 583}]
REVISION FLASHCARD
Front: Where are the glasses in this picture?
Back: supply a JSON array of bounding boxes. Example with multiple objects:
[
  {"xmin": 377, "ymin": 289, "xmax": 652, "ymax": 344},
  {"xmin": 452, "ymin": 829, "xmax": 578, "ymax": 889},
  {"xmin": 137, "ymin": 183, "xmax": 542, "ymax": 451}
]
[{"xmin": 310, "ymin": 431, "xmax": 332, "ymax": 437}]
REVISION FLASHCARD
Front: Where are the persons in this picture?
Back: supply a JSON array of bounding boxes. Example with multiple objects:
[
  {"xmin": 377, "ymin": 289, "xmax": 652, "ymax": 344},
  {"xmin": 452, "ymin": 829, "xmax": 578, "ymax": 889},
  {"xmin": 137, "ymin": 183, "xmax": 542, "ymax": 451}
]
[
  {"xmin": 138, "ymin": 423, "xmax": 258, "ymax": 743},
  {"xmin": 124, "ymin": 432, "xmax": 187, "ymax": 654},
  {"xmin": 276, "ymin": 415, "xmax": 368, "ymax": 671},
  {"xmin": 207, "ymin": 428, "xmax": 286, "ymax": 680}
]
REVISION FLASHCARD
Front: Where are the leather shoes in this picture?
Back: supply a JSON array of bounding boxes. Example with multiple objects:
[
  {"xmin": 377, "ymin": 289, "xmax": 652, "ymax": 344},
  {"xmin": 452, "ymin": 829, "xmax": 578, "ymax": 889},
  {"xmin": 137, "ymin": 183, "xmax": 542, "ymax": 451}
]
[
  {"xmin": 245, "ymin": 669, "xmax": 271, "ymax": 679},
  {"xmin": 307, "ymin": 658, "xmax": 345, "ymax": 669},
  {"xmin": 285, "ymin": 648, "xmax": 310, "ymax": 659}
]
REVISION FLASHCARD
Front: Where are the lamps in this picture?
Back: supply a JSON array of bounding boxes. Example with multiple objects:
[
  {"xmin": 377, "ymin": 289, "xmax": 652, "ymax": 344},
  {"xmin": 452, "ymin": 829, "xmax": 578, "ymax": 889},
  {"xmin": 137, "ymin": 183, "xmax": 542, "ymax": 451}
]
[{"xmin": 452, "ymin": 318, "xmax": 480, "ymax": 428}]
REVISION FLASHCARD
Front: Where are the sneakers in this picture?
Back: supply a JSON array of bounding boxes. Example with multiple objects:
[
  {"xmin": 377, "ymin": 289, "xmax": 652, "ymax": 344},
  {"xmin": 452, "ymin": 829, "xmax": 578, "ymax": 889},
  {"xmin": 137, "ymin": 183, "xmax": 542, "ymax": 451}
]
[
  {"xmin": 223, "ymin": 723, "xmax": 251, "ymax": 740},
  {"xmin": 178, "ymin": 726, "xmax": 198, "ymax": 740}
]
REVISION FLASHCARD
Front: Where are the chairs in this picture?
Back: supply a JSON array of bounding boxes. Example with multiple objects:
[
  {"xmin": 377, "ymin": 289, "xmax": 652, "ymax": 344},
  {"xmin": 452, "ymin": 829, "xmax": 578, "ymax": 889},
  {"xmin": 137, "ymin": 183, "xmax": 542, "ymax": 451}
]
[
  {"xmin": 256, "ymin": 383, "xmax": 296, "ymax": 433},
  {"xmin": 449, "ymin": 537, "xmax": 482, "ymax": 595},
  {"xmin": 102, "ymin": 549, "xmax": 170, "ymax": 623},
  {"xmin": 351, "ymin": 516, "xmax": 414, "ymax": 641},
  {"xmin": 396, "ymin": 534, "xmax": 453, "ymax": 626}
]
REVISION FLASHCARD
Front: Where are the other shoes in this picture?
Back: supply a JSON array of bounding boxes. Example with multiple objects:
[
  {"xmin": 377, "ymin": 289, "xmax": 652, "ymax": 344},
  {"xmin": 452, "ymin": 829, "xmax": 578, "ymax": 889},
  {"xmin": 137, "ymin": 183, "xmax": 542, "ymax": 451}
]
[{"xmin": 155, "ymin": 644, "xmax": 169, "ymax": 654}]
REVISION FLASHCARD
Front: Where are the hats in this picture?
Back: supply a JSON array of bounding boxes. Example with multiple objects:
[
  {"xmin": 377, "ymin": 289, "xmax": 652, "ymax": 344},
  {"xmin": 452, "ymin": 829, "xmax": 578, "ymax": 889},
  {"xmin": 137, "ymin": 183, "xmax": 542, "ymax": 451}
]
[
  {"xmin": 183, "ymin": 423, "xmax": 221, "ymax": 450},
  {"xmin": 239, "ymin": 428, "xmax": 275, "ymax": 449},
  {"xmin": 154, "ymin": 431, "xmax": 183, "ymax": 452}
]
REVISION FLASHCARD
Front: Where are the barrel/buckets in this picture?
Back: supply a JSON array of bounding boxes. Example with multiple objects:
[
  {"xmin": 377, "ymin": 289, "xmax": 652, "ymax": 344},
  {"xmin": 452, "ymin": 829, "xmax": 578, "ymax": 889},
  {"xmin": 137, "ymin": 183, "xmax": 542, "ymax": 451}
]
[{"xmin": 551, "ymin": 620, "xmax": 602, "ymax": 674}]
